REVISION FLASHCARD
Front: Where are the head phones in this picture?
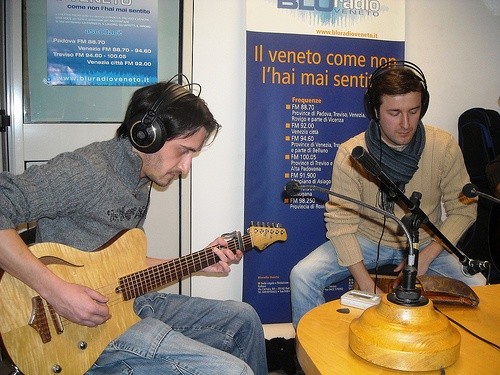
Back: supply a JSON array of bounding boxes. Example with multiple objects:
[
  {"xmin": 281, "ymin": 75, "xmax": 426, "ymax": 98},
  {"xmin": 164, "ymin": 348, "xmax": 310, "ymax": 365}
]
[
  {"xmin": 127, "ymin": 74, "xmax": 202, "ymax": 153},
  {"xmin": 363, "ymin": 61, "xmax": 429, "ymax": 121}
]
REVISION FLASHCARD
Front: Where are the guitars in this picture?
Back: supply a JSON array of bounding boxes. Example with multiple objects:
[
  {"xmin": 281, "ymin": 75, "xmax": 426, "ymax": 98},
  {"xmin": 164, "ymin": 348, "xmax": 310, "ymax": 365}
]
[{"xmin": 0, "ymin": 221, "xmax": 288, "ymax": 375}]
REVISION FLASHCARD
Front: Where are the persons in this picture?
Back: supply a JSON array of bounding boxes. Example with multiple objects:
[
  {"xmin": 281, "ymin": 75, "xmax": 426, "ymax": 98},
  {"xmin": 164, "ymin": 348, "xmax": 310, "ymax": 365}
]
[
  {"xmin": 291, "ymin": 69, "xmax": 487, "ymax": 327},
  {"xmin": 0, "ymin": 82, "xmax": 269, "ymax": 375}
]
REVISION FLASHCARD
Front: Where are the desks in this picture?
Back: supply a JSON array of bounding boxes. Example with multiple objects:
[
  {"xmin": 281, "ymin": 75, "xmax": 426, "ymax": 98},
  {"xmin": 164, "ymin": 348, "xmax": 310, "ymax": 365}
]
[{"xmin": 296, "ymin": 284, "xmax": 500, "ymax": 375}]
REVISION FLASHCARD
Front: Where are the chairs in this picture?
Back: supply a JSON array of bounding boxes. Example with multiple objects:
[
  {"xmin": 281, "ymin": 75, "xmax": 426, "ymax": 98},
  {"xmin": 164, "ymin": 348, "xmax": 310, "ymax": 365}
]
[{"xmin": 457, "ymin": 108, "xmax": 500, "ymax": 284}]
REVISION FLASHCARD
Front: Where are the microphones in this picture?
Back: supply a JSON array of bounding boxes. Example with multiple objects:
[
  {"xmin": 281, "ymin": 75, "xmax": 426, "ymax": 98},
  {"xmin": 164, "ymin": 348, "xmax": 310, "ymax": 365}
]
[
  {"xmin": 461, "ymin": 259, "xmax": 490, "ymax": 277},
  {"xmin": 462, "ymin": 183, "xmax": 500, "ymax": 204},
  {"xmin": 286, "ymin": 181, "xmax": 431, "ymax": 305}
]
